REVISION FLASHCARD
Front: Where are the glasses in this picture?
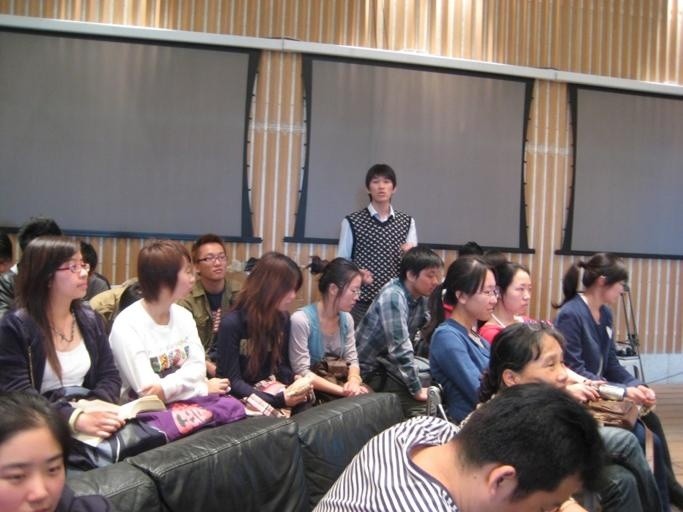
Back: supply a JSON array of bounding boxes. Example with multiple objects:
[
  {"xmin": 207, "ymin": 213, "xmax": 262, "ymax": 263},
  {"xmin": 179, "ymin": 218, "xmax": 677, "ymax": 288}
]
[
  {"xmin": 195, "ymin": 255, "xmax": 230, "ymax": 263},
  {"xmin": 53, "ymin": 261, "xmax": 92, "ymax": 274}
]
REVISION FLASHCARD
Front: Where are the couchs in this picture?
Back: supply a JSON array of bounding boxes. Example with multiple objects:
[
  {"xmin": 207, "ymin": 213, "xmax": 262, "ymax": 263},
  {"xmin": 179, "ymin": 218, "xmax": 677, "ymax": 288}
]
[{"xmin": 1, "ymin": 387, "xmax": 408, "ymax": 512}]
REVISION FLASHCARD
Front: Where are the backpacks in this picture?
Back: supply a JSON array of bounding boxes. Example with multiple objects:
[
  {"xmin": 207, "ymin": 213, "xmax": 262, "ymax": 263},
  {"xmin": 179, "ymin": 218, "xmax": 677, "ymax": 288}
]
[
  {"xmin": 49, "ymin": 384, "xmax": 169, "ymax": 472},
  {"xmin": 577, "ymin": 394, "xmax": 639, "ymax": 429},
  {"xmin": 310, "ymin": 351, "xmax": 373, "ymax": 403}
]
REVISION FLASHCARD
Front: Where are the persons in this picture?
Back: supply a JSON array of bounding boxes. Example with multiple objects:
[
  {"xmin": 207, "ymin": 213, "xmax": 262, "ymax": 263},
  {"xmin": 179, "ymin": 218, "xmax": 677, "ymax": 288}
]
[
  {"xmin": 214, "ymin": 251, "xmax": 316, "ymax": 415},
  {"xmin": 421, "ymin": 254, "xmax": 664, "ymax": 510},
  {"xmin": 2, "ymin": 220, "xmax": 125, "ymax": 438},
  {"xmin": 87, "ymin": 277, "xmax": 146, "ymax": 328},
  {"xmin": 460, "ymin": 321, "xmax": 584, "ymax": 511},
  {"xmin": 481, "ymin": 257, "xmax": 682, "ymax": 511},
  {"xmin": 312, "ymin": 383, "xmax": 606, "ymax": 510},
  {"xmin": 175, "ymin": 234, "xmax": 249, "ymax": 374},
  {"xmin": 1, "ymin": 391, "xmax": 115, "ymax": 511},
  {"xmin": 106, "ymin": 240, "xmax": 231, "ymax": 408},
  {"xmin": 355, "ymin": 245, "xmax": 445, "ymax": 416},
  {"xmin": 458, "ymin": 241, "xmax": 483, "ymax": 256},
  {"xmin": 287, "ymin": 256, "xmax": 374, "ymax": 404},
  {"xmin": 337, "ymin": 164, "xmax": 417, "ymax": 328}
]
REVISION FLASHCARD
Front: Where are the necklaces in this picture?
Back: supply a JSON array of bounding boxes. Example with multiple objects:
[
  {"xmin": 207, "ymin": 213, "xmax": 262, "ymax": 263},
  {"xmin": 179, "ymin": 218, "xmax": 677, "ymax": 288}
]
[{"xmin": 46, "ymin": 308, "xmax": 76, "ymax": 343}]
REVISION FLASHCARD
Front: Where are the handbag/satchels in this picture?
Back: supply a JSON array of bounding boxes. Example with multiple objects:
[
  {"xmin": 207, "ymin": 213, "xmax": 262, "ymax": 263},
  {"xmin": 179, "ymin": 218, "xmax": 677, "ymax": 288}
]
[
  {"xmin": 134, "ymin": 389, "xmax": 247, "ymax": 442},
  {"xmin": 372, "ymin": 354, "xmax": 434, "ymax": 389},
  {"xmin": 632, "ymin": 395, "xmax": 656, "ymax": 416},
  {"xmin": 239, "ymin": 377, "xmax": 294, "ymax": 423}
]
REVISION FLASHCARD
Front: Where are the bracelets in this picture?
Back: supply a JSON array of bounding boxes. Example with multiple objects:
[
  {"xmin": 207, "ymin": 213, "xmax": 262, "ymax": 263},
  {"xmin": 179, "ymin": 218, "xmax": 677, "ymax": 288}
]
[{"xmin": 66, "ymin": 409, "xmax": 84, "ymax": 433}]
